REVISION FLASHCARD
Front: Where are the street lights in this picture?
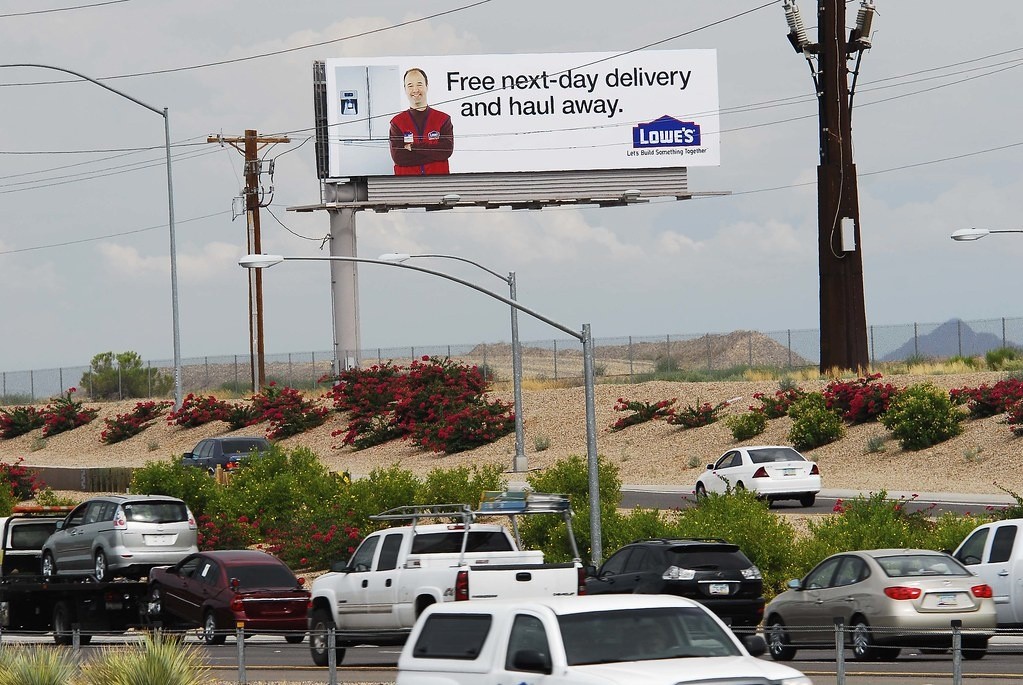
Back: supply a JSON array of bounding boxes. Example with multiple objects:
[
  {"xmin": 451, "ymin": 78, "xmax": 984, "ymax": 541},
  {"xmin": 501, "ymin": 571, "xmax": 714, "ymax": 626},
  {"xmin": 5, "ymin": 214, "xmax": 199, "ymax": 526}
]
[
  {"xmin": 238, "ymin": 251, "xmax": 602, "ymax": 568},
  {"xmin": 379, "ymin": 251, "xmax": 528, "ymax": 473}
]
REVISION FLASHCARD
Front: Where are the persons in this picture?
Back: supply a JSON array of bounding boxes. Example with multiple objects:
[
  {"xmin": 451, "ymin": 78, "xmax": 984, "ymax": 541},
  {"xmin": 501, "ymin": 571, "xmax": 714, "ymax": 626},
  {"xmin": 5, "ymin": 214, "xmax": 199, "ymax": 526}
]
[
  {"xmin": 636, "ymin": 623, "xmax": 667, "ymax": 657},
  {"xmin": 389, "ymin": 69, "xmax": 454, "ymax": 174}
]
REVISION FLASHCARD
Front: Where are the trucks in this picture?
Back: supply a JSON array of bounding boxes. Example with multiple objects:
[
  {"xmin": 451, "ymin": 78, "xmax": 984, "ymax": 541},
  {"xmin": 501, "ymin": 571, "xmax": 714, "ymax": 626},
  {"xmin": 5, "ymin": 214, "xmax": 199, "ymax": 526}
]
[{"xmin": 0, "ymin": 506, "xmax": 147, "ymax": 645}]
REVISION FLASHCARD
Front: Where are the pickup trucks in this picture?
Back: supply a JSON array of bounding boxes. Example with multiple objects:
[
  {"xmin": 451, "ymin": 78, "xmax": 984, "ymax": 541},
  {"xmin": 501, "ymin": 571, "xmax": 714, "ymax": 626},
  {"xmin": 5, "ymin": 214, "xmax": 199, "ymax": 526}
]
[
  {"xmin": 308, "ymin": 523, "xmax": 589, "ymax": 667},
  {"xmin": 919, "ymin": 519, "xmax": 1023, "ymax": 655}
]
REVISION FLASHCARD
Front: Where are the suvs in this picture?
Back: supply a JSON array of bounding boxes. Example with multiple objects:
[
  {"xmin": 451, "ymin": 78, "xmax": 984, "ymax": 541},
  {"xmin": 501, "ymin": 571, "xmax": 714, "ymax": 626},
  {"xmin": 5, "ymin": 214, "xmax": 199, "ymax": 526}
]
[
  {"xmin": 396, "ymin": 594, "xmax": 812, "ymax": 685},
  {"xmin": 584, "ymin": 537, "xmax": 766, "ymax": 637},
  {"xmin": 41, "ymin": 494, "xmax": 199, "ymax": 580}
]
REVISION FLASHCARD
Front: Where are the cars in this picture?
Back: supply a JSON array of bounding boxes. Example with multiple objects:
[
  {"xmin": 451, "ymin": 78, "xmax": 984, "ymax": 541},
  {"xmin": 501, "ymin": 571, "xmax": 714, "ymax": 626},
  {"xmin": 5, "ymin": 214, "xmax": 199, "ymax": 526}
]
[
  {"xmin": 147, "ymin": 549, "xmax": 310, "ymax": 645},
  {"xmin": 762, "ymin": 549, "xmax": 999, "ymax": 663},
  {"xmin": 179, "ymin": 437, "xmax": 288, "ymax": 476},
  {"xmin": 695, "ymin": 446, "xmax": 821, "ymax": 508}
]
